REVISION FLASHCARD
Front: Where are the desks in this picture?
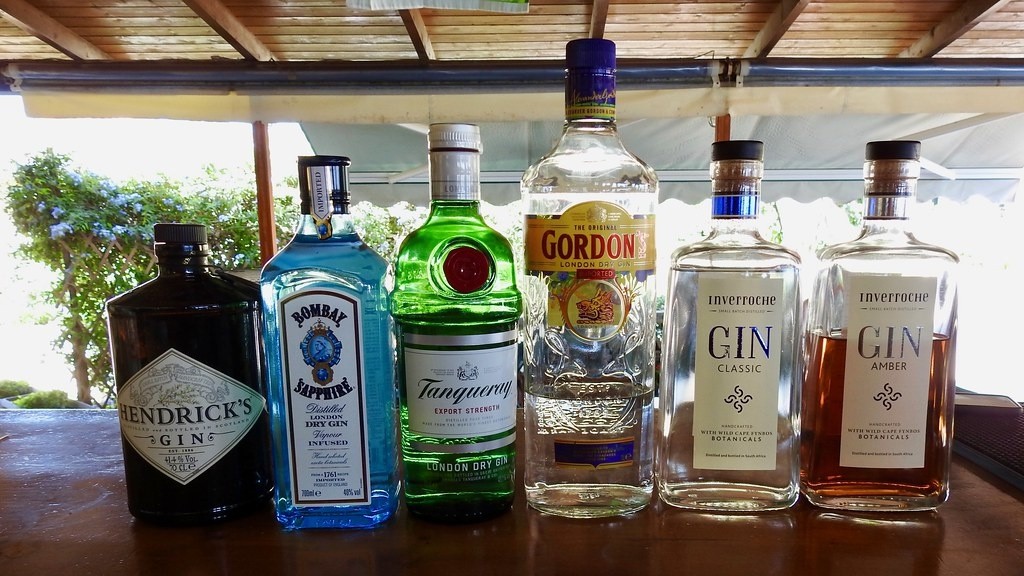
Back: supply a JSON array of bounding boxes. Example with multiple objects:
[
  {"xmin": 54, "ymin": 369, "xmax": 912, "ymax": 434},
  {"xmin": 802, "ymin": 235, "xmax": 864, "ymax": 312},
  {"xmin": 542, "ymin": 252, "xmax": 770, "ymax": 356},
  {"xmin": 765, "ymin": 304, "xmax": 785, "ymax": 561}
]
[{"xmin": 0, "ymin": 407, "xmax": 1024, "ymax": 576}]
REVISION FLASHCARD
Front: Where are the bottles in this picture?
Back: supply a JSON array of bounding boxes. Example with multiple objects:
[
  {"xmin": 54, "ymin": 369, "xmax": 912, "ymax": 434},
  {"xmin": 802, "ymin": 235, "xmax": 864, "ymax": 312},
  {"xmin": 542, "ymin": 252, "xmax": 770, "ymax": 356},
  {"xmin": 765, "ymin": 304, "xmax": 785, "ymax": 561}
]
[
  {"xmin": 661, "ymin": 140, "xmax": 832, "ymax": 515},
  {"xmin": 800, "ymin": 140, "xmax": 959, "ymax": 512},
  {"xmin": 521, "ymin": 36, "xmax": 660, "ymax": 522},
  {"xmin": 259, "ymin": 155, "xmax": 400, "ymax": 529},
  {"xmin": 387, "ymin": 122, "xmax": 520, "ymax": 509},
  {"xmin": 105, "ymin": 223, "xmax": 277, "ymax": 527}
]
